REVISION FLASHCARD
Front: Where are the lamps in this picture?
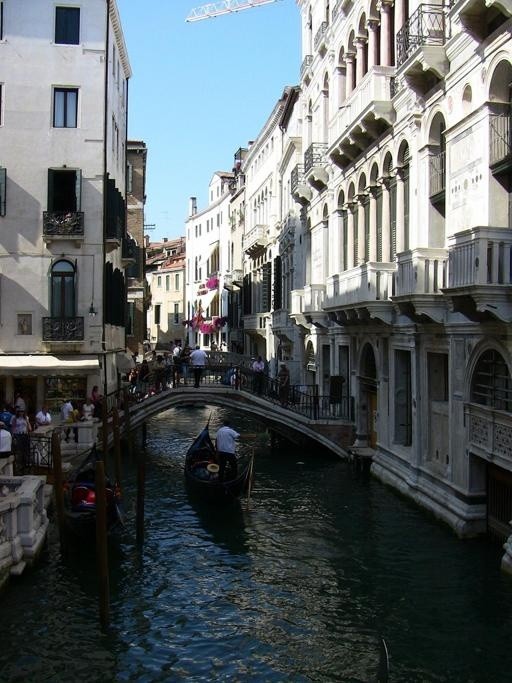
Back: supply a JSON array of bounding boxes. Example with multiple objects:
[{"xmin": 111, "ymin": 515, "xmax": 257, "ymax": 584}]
[{"xmin": 89, "ymin": 304, "xmax": 96, "ymax": 320}]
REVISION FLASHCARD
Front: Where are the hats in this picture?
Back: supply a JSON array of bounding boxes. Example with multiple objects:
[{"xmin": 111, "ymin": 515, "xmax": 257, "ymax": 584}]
[
  {"xmin": 17, "ymin": 407, "xmax": 25, "ymax": 412},
  {"xmin": 280, "ymin": 362, "xmax": 288, "ymax": 366},
  {"xmin": 207, "ymin": 464, "xmax": 219, "ymax": 473}
]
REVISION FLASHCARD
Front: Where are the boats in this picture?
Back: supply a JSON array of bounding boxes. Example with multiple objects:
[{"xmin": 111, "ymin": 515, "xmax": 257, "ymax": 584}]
[
  {"xmin": 185, "ymin": 409, "xmax": 254, "ymax": 506},
  {"xmin": 60, "ymin": 444, "xmax": 126, "ymax": 533}
]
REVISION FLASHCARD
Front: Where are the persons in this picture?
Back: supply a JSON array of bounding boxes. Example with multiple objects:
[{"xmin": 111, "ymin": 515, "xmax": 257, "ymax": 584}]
[
  {"xmin": 251, "ymin": 355, "xmax": 265, "ymax": 394},
  {"xmin": 213, "ymin": 417, "xmax": 241, "ymax": 477},
  {"xmin": 0, "ymin": 383, "xmax": 100, "ymax": 459},
  {"xmin": 274, "ymin": 361, "xmax": 292, "ymax": 408},
  {"xmin": 124, "ymin": 335, "xmax": 221, "ymax": 400}
]
